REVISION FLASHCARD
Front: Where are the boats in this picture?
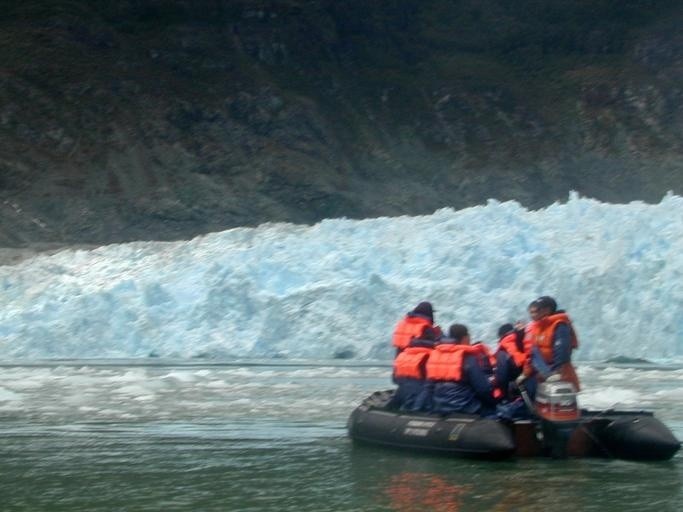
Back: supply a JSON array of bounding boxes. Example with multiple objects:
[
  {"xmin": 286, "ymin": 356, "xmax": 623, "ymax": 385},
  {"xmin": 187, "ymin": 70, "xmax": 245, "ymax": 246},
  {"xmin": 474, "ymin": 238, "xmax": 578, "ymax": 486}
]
[{"xmin": 345, "ymin": 385, "xmax": 683, "ymax": 466}]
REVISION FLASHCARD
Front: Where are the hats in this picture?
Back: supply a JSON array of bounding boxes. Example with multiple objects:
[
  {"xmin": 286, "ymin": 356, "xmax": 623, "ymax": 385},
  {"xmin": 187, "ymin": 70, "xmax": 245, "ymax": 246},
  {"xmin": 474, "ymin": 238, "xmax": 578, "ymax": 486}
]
[
  {"xmin": 498, "ymin": 323, "xmax": 514, "ymax": 335},
  {"xmin": 537, "ymin": 296, "xmax": 557, "ymax": 311},
  {"xmin": 425, "ymin": 328, "xmax": 435, "ymax": 338},
  {"xmin": 449, "ymin": 325, "xmax": 466, "ymax": 338},
  {"xmin": 415, "ymin": 302, "xmax": 437, "ymax": 314}
]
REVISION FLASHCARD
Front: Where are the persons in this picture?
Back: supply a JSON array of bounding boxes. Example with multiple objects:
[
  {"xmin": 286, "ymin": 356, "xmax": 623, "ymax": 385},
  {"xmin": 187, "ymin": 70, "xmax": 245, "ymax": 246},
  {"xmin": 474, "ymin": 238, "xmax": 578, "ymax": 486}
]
[
  {"xmin": 382, "ymin": 301, "xmax": 436, "ymax": 413},
  {"xmin": 390, "ymin": 326, "xmax": 441, "ymax": 413},
  {"xmin": 498, "ymin": 295, "xmax": 580, "ymax": 418},
  {"xmin": 491, "ymin": 322, "xmax": 524, "ymax": 390},
  {"xmin": 511, "ymin": 300, "xmax": 541, "ymax": 372},
  {"xmin": 408, "ymin": 324, "xmax": 499, "ymax": 420}
]
